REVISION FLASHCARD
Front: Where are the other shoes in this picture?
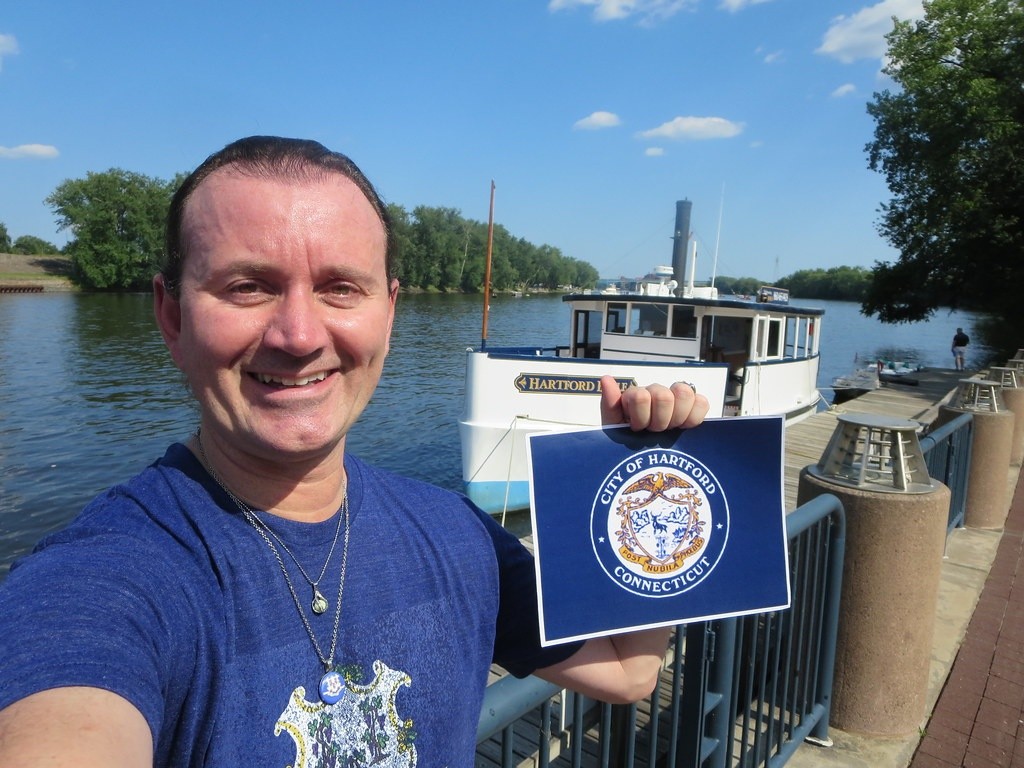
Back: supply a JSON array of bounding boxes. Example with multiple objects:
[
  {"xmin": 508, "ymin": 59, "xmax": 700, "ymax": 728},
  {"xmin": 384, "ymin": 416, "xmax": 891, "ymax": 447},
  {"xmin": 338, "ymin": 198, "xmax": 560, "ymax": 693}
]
[{"xmin": 955, "ymin": 368, "xmax": 965, "ymax": 371}]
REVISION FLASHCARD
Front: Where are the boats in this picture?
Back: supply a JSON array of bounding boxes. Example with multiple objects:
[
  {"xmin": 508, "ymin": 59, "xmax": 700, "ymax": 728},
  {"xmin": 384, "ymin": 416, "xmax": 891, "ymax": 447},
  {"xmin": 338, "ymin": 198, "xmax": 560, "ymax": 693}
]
[{"xmin": 455, "ymin": 168, "xmax": 825, "ymax": 527}]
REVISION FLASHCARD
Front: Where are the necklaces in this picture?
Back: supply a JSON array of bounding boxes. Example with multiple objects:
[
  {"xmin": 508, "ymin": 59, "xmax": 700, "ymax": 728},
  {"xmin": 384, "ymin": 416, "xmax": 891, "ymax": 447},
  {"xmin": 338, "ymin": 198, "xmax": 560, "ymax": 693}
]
[{"xmin": 198, "ymin": 429, "xmax": 349, "ymax": 705}]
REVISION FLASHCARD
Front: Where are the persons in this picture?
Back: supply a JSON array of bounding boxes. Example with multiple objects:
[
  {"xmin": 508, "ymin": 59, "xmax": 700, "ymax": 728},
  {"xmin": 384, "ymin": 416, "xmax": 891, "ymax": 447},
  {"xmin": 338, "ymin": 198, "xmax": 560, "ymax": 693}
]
[
  {"xmin": 0, "ymin": 138, "xmax": 711, "ymax": 768},
  {"xmin": 951, "ymin": 328, "xmax": 969, "ymax": 370}
]
